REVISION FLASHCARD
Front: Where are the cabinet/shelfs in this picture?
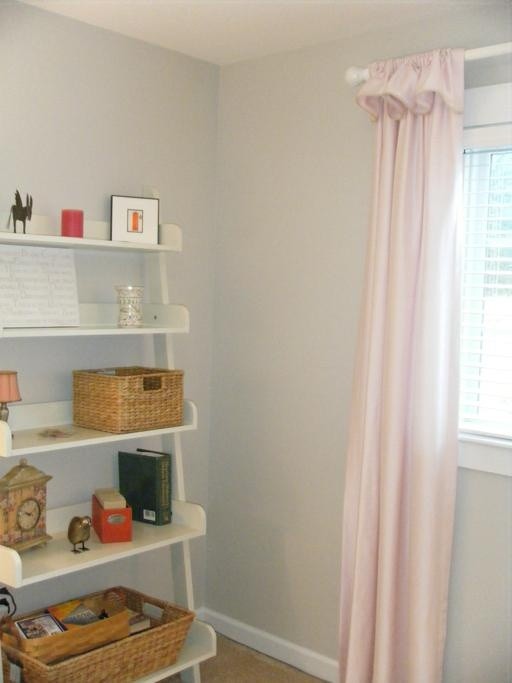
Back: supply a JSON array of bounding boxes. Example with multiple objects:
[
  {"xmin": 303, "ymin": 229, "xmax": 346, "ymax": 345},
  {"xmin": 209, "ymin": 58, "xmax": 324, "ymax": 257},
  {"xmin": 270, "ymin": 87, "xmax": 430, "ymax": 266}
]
[{"xmin": 1, "ymin": 212, "xmax": 219, "ymax": 683}]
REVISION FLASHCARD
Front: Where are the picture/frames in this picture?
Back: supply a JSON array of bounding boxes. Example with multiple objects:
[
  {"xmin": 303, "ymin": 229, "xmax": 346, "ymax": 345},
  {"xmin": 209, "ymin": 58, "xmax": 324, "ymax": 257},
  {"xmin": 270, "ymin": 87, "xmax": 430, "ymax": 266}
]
[{"xmin": 109, "ymin": 194, "xmax": 160, "ymax": 245}]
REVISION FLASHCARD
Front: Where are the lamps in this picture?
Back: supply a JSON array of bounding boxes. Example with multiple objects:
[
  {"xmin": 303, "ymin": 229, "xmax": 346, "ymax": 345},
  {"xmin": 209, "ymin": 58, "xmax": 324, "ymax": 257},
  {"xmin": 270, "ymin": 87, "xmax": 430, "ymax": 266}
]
[{"xmin": 1, "ymin": 370, "xmax": 23, "ymax": 440}]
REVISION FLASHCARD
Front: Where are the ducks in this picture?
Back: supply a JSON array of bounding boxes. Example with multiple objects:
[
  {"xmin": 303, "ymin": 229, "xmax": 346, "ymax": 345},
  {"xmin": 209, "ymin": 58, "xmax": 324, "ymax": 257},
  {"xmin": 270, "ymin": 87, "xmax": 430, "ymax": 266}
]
[{"xmin": 67, "ymin": 516, "xmax": 93, "ymax": 553}]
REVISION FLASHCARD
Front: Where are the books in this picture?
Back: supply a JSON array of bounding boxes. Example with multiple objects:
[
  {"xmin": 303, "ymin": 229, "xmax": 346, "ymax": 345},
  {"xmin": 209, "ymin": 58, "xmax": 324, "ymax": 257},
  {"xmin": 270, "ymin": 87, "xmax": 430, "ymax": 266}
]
[
  {"xmin": 13, "ymin": 593, "xmax": 152, "ymax": 651},
  {"xmin": 118, "ymin": 446, "xmax": 173, "ymax": 526}
]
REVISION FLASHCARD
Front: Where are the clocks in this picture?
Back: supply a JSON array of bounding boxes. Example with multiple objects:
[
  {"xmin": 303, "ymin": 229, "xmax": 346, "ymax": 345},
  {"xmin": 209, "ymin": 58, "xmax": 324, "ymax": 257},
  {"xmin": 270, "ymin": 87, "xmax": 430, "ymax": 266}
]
[{"xmin": 0, "ymin": 458, "xmax": 54, "ymax": 553}]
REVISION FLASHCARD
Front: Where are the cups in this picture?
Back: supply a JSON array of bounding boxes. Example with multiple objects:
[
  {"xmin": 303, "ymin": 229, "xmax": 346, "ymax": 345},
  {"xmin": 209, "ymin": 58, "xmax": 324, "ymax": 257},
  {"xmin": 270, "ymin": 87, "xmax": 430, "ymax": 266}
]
[{"xmin": 114, "ymin": 283, "xmax": 146, "ymax": 329}]
